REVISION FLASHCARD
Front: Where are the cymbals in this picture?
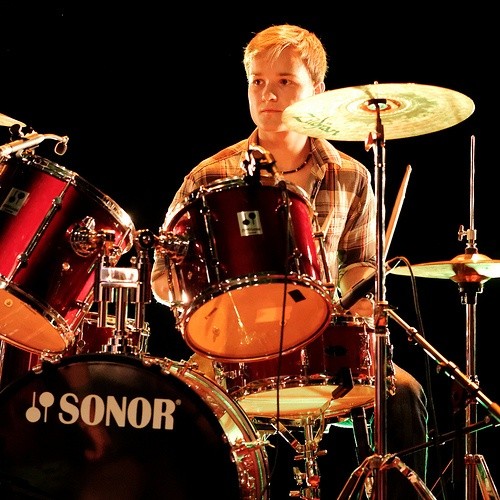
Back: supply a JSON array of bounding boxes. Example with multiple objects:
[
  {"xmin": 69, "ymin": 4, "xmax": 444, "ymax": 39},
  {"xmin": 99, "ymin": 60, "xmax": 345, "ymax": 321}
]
[
  {"xmin": 389, "ymin": 255, "xmax": 500, "ymax": 279},
  {"xmin": 282, "ymin": 84, "xmax": 475, "ymax": 141}
]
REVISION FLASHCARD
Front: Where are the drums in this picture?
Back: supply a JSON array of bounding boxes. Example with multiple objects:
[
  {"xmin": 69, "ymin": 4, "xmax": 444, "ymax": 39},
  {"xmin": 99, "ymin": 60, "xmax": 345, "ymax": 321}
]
[
  {"xmin": 0, "ymin": 155, "xmax": 135, "ymax": 358},
  {"xmin": 164, "ymin": 176, "xmax": 336, "ymax": 363},
  {"xmin": 222, "ymin": 282, "xmax": 376, "ymax": 422},
  {"xmin": 0, "ymin": 353, "xmax": 270, "ymax": 500}
]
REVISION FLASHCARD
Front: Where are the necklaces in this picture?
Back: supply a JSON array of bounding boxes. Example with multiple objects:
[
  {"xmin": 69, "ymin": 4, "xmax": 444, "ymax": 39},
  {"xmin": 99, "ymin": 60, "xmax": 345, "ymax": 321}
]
[{"xmin": 277, "ymin": 149, "xmax": 313, "ymax": 176}]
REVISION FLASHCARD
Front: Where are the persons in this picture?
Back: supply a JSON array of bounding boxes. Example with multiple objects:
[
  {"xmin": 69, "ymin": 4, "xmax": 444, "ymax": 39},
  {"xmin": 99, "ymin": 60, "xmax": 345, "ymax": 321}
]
[{"xmin": 149, "ymin": 23, "xmax": 429, "ymax": 500}]
